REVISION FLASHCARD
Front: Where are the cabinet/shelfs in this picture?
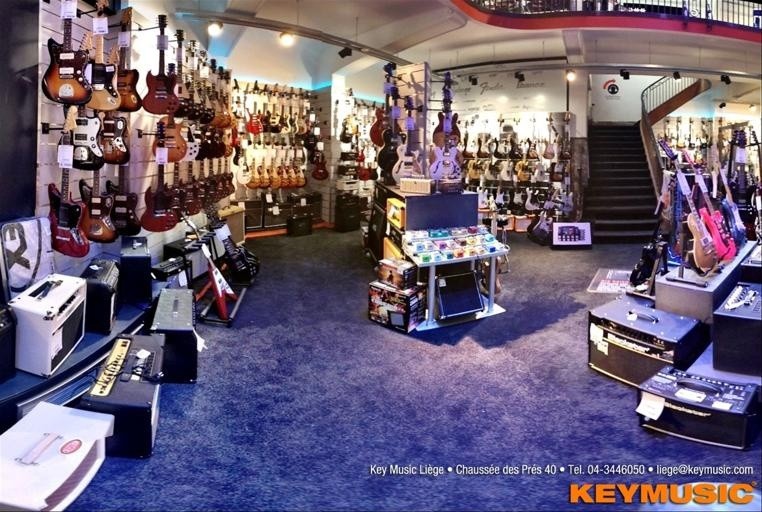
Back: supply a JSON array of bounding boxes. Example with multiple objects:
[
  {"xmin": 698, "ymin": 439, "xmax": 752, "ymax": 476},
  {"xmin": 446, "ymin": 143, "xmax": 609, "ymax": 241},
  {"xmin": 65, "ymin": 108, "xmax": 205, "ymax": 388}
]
[{"xmin": 365, "ymin": 179, "xmax": 479, "ymax": 274}]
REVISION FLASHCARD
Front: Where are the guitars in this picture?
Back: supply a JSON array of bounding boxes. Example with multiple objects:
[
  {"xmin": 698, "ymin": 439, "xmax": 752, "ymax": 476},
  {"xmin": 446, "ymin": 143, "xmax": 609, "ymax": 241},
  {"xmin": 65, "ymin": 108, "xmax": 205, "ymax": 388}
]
[
  {"xmin": 41, "ymin": 1, "xmax": 141, "ymax": 258},
  {"xmin": 142, "ymin": 15, "xmax": 234, "ymax": 232},
  {"xmin": 181, "ymin": 197, "xmax": 260, "ymax": 319},
  {"xmin": 233, "ymin": 78, "xmax": 328, "ymax": 190},
  {"xmin": 629, "ymin": 129, "xmax": 762, "ymax": 285},
  {"xmin": 465, "ymin": 115, "xmax": 575, "ymax": 246},
  {"xmin": 370, "ymin": 63, "xmax": 464, "ymax": 184},
  {"xmin": 337, "ymin": 99, "xmax": 385, "ymax": 180}
]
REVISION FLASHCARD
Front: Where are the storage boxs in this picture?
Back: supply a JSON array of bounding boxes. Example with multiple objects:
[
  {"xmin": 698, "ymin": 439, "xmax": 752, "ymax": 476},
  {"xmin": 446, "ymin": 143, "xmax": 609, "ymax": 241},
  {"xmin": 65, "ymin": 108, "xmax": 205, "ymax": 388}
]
[
  {"xmin": 367, "ymin": 258, "xmax": 429, "ymax": 334},
  {"xmin": 174, "ymin": 0, "xmax": 414, "ymax": 72}
]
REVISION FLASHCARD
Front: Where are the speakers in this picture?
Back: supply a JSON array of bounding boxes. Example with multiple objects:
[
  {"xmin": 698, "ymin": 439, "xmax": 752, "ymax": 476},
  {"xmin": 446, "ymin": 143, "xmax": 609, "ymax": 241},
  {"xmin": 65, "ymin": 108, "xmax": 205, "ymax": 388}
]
[
  {"xmin": 587, "ymin": 240, "xmax": 762, "ymax": 451},
  {"xmin": 287, "ymin": 214, "xmax": 312, "ymax": 236},
  {"xmin": 0, "ymin": 236, "xmax": 209, "ymax": 512},
  {"xmin": 335, "ymin": 195, "xmax": 361, "ymax": 233}
]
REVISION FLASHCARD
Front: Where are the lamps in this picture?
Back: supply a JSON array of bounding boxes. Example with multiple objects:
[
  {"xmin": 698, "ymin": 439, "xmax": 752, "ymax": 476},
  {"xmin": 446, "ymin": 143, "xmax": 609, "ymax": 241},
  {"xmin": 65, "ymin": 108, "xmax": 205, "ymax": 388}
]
[{"xmin": 456, "ymin": 67, "xmax": 761, "ymax": 85}]
